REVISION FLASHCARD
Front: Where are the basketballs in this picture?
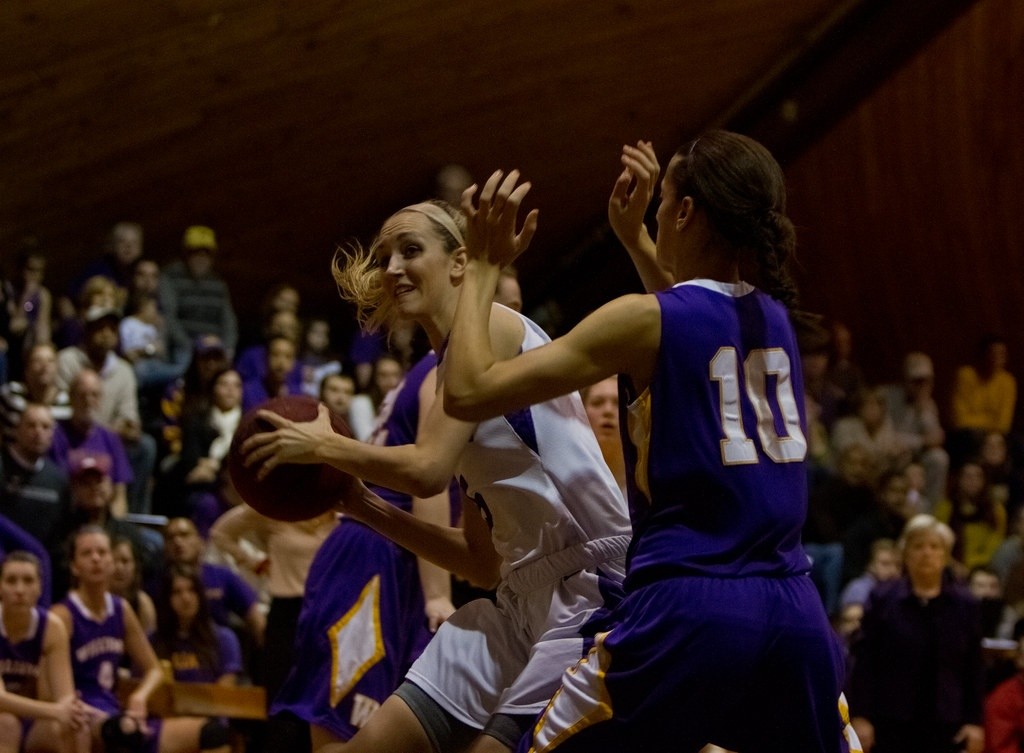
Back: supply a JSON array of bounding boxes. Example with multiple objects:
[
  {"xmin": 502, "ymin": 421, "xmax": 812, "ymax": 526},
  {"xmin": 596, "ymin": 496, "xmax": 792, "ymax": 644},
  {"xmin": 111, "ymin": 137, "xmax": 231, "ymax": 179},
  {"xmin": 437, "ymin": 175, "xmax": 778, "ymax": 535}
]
[{"xmin": 228, "ymin": 393, "xmax": 356, "ymax": 523}]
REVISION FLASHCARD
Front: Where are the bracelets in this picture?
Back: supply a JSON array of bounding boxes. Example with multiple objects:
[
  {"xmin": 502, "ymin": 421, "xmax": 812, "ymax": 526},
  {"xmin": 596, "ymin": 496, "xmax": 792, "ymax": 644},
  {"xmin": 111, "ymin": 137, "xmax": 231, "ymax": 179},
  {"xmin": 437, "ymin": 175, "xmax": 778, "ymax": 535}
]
[{"xmin": 252, "ymin": 560, "xmax": 271, "ymax": 579}]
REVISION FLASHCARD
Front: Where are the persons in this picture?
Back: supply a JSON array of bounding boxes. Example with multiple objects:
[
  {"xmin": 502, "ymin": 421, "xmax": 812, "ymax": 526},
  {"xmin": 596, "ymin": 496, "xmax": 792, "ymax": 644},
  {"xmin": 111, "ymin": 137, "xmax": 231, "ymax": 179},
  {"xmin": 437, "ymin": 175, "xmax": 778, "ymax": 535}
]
[{"xmin": 0, "ymin": 132, "xmax": 1023, "ymax": 753}]
